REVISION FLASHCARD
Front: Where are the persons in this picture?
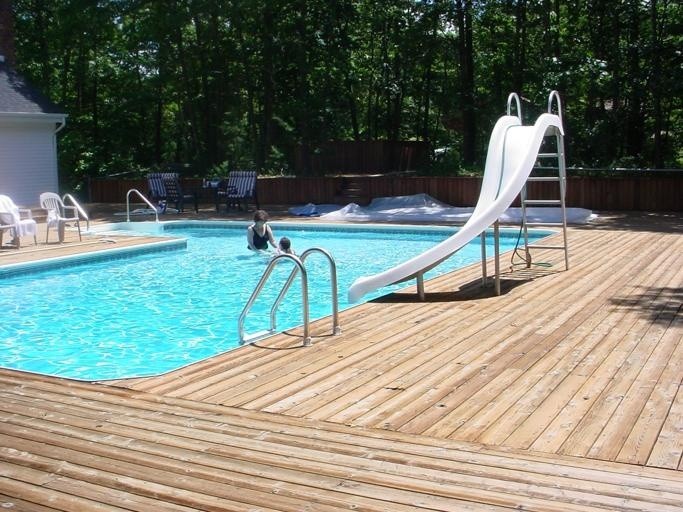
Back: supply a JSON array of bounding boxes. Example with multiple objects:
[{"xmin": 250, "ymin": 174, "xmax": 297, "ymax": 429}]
[
  {"xmin": 258, "ymin": 237, "xmax": 296, "ymax": 258},
  {"xmin": 247, "ymin": 209, "xmax": 279, "ymax": 255}
]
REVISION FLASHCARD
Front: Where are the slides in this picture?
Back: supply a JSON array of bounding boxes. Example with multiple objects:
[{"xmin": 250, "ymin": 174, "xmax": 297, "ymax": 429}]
[{"xmin": 347, "ymin": 112, "xmax": 566, "ymax": 303}]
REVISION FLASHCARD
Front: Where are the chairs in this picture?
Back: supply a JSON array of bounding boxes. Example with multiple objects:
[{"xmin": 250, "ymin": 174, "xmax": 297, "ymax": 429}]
[
  {"xmin": 0, "ymin": 195, "xmax": 37, "ymax": 251},
  {"xmin": 145, "ymin": 170, "xmax": 260, "ymax": 216},
  {"xmin": 39, "ymin": 191, "xmax": 81, "ymax": 246}
]
